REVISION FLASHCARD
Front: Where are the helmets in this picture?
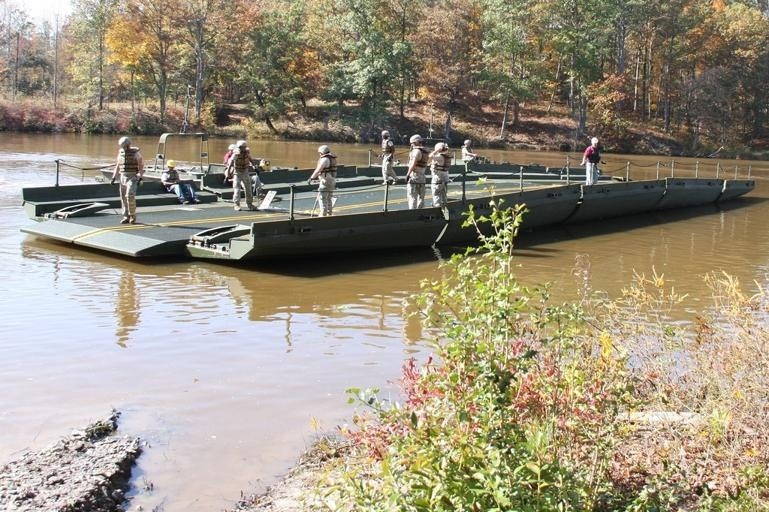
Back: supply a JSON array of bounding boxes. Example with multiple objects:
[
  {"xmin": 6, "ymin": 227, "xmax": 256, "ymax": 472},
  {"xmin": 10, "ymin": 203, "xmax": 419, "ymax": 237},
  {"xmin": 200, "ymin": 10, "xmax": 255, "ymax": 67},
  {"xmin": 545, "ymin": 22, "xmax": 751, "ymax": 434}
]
[
  {"xmin": 118, "ymin": 136, "xmax": 131, "ymax": 149},
  {"xmin": 435, "ymin": 142, "xmax": 447, "ymax": 151},
  {"xmin": 228, "ymin": 140, "xmax": 249, "ymax": 151},
  {"xmin": 318, "ymin": 145, "xmax": 329, "ymax": 154},
  {"xmin": 463, "ymin": 139, "xmax": 472, "ymax": 144},
  {"xmin": 409, "ymin": 134, "xmax": 424, "ymax": 143},
  {"xmin": 381, "ymin": 130, "xmax": 390, "ymax": 137},
  {"xmin": 167, "ymin": 160, "xmax": 176, "ymax": 168}
]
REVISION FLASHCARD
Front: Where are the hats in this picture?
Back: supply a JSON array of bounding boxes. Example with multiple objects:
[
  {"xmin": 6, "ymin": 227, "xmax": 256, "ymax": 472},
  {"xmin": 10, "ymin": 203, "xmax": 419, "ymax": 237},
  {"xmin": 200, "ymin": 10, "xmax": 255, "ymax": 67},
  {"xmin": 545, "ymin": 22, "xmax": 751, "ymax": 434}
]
[{"xmin": 591, "ymin": 137, "xmax": 599, "ymax": 143}]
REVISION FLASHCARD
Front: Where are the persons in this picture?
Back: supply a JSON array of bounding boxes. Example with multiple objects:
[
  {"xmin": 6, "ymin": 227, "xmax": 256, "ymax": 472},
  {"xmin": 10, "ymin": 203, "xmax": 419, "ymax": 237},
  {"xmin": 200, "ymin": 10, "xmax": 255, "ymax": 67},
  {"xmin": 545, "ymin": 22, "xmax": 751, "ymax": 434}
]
[
  {"xmin": 109, "ymin": 136, "xmax": 146, "ymax": 225},
  {"xmin": 405, "ymin": 133, "xmax": 427, "ymax": 210},
  {"xmin": 379, "ymin": 129, "xmax": 400, "ymax": 186},
  {"xmin": 462, "ymin": 139, "xmax": 478, "ymax": 165},
  {"xmin": 223, "ymin": 140, "xmax": 263, "ymax": 211},
  {"xmin": 223, "ymin": 144, "xmax": 238, "ymax": 182},
  {"xmin": 426, "ymin": 142, "xmax": 451, "ymax": 208},
  {"xmin": 307, "ymin": 145, "xmax": 336, "ymax": 217},
  {"xmin": 579, "ymin": 137, "xmax": 599, "ymax": 186},
  {"xmin": 159, "ymin": 159, "xmax": 202, "ymax": 205}
]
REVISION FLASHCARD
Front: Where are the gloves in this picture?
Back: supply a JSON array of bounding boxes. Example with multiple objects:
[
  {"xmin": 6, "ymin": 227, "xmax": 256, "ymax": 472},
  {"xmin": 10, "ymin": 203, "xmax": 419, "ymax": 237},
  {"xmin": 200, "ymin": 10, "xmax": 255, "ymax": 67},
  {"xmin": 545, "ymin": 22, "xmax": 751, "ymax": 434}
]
[
  {"xmin": 111, "ymin": 177, "xmax": 115, "ymax": 186},
  {"xmin": 254, "ymin": 168, "xmax": 260, "ymax": 174},
  {"xmin": 136, "ymin": 177, "xmax": 142, "ymax": 186},
  {"xmin": 307, "ymin": 178, "xmax": 312, "ymax": 185},
  {"xmin": 405, "ymin": 175, "xmax": 409, "ymax": 182}
]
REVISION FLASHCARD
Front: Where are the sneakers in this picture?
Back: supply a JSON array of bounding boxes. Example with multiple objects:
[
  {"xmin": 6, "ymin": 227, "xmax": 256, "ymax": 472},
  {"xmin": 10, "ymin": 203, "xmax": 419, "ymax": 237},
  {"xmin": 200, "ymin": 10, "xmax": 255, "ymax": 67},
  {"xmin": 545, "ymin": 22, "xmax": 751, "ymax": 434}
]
[
  {"xmin": 120, "ymin": 219, "xmax": 129, "ymax": 224},
  {"xmin": 234, "ymin": 204, "xmax": 242, "ymax": 211},
  {"xmin": 382, "ymin": 180, "xmax": 390, "ymax": 185},
  {"xmin": 247, "ymin": 204, "xmax": 258, "ymax": 210},
  {"xmin": 391, "ymin": 177, "xmax": 399, "ymax": 185},
  {"xmin": 183, "ymin": 201, "xmax": 189, "ymax": 204},
  {"xmin": 194, "ymin": 199, "xmax": 202, "ymax": 204},
  {"xmin": 318, "ymin": 211, "xmax": 333, "ymax": 218},
  {"xmin": 129, "ymin": 214, "xmax": 137, "ymax": 223}
]
